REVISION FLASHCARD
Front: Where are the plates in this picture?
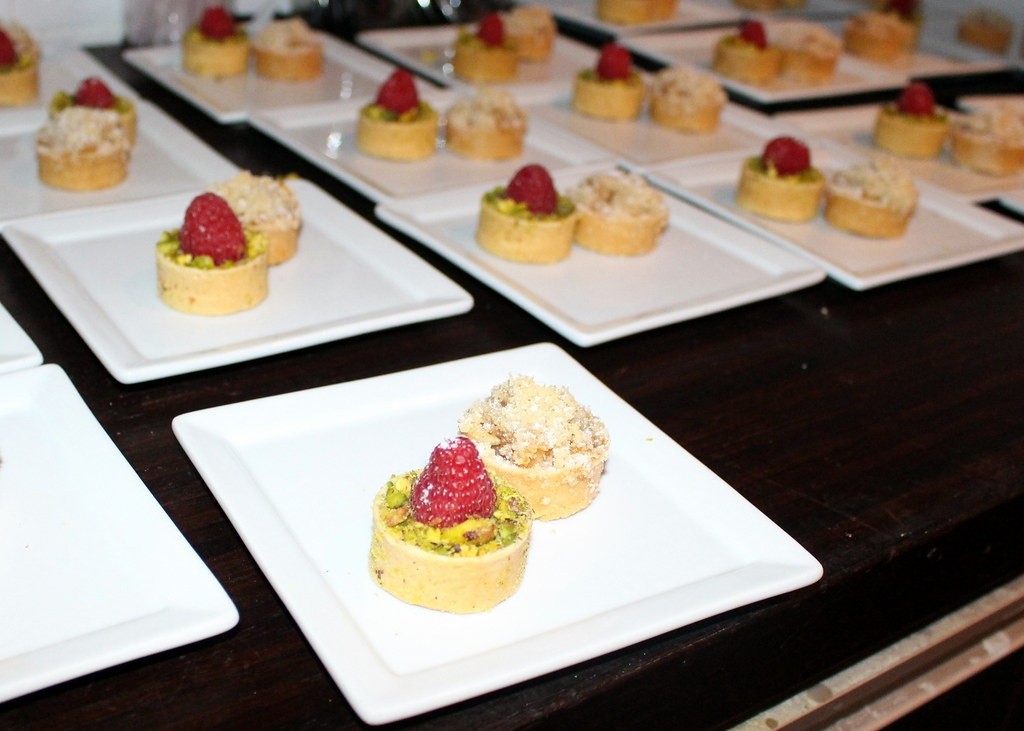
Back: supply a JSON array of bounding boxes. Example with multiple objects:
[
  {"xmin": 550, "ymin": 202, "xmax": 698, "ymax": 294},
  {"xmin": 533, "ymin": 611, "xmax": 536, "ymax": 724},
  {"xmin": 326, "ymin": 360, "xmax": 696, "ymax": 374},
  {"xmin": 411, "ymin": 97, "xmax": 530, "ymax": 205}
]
[
  {"xmin": 773, "ymin": 95, "xmax": 1024, "ymax": 215},
  {"xmin": 374, "ymin": 167, "xmax": 826, "ymax": 348},
  {"xmin": 122, "ymin": 31, "xmax": 441, "ymax": 123},
  {"xmin": 642, "ymin": 146, "xmax": 1024, "ymax": 290},
  {"xmin": 1, "ymin": 300, "xmax": 240, "ymax": 704},
  {"xmin": 517, "ymin": 0, "xmax": 1024, "ymax": 103},
  {"xmin": 0, "ymin": 176, "xmax": 473, "ymax": 383},
  {"xmin": 354, "ymin": 21, "xmax": 775, "ymax": 175},
  {"xmin": 171, "ymin": 342, "xmax": 823, "ymax": 726},
  {"xmin": 250, "ymin": 91, "xmax": 623, "ymax": 203},
  {"xmin": 0, "ymin": 48, "xmax": 242, "ymax": 229}
]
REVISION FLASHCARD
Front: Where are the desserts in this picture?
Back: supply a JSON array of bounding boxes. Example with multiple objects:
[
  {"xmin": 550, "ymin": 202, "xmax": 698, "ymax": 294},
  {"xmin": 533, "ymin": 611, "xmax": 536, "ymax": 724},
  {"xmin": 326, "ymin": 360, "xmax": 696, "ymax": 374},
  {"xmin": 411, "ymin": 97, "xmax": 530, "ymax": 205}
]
[
  {"xmin": 369, "ymin": 368, "xmax": 608, "ymax": 613},
  {"xmin": 0, "ymin": 0, "xmax": 1024, "ymax": 316}
]
